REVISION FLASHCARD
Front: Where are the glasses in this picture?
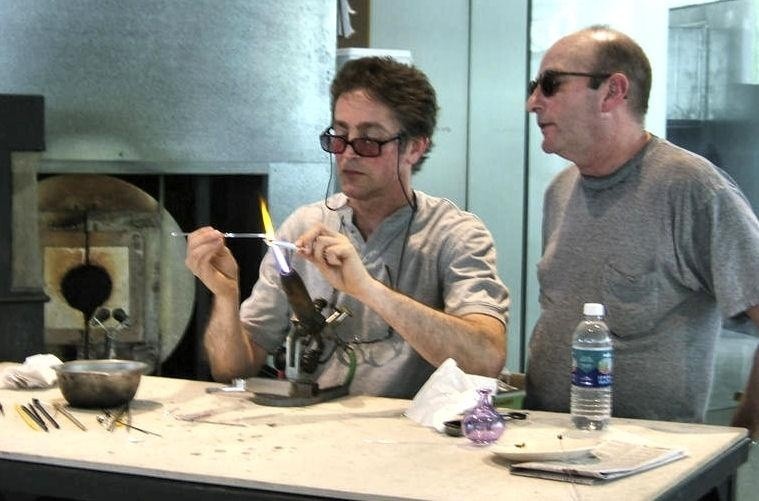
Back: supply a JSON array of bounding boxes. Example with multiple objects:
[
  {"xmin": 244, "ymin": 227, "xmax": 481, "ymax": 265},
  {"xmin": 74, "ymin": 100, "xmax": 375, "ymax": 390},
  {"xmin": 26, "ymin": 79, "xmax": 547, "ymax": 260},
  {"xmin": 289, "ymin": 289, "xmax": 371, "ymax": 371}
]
[
  {"xmin": 528, "ymin": 72, "xmax": 612, "ymax": 97},
  {"xmin": 319, "ymin": 125, "xmax": 407, "ymax": 157}
]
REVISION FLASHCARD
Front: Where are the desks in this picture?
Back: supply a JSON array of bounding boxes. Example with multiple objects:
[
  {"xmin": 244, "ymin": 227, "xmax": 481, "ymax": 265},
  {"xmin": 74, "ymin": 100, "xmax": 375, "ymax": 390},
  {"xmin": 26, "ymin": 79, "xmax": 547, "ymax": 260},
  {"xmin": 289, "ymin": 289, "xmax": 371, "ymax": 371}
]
[{"xmin": 0, "ymin": 362, "xmax": 752, "ymax": 501}]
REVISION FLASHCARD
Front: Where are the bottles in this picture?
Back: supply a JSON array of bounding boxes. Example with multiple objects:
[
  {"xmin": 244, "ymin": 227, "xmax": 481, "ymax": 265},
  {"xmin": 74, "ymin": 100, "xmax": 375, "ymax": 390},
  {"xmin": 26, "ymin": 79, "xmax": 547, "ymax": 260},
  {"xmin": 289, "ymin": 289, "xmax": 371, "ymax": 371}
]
[
  {"xmin": 572, "ymin": 303, "xmax": 613, "ymax": 428},
  {"xmin": 462, "ymin": 387, "xmax": 505, "ymax": 448}
]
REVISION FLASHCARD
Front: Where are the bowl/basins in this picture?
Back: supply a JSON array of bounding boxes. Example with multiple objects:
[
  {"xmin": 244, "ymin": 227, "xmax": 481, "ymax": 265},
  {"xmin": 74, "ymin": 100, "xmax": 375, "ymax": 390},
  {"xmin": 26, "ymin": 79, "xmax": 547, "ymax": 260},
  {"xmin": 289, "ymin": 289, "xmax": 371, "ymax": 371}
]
[{"xmin": 51, "ymin": 359, "xmax": 148, "ymax": 410}]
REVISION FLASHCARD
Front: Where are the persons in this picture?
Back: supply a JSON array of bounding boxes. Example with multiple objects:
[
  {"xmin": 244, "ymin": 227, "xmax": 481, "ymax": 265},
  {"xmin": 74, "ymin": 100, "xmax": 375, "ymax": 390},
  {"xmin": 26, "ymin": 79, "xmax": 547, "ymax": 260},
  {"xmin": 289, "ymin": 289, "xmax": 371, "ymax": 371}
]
[
  {"xmin": 520, "ymin": 23, "xmax": 759, "ymax": 500},
  {"xmin": 184, "ymin": 54, "xmax": 509, "ymax": 401}
]
